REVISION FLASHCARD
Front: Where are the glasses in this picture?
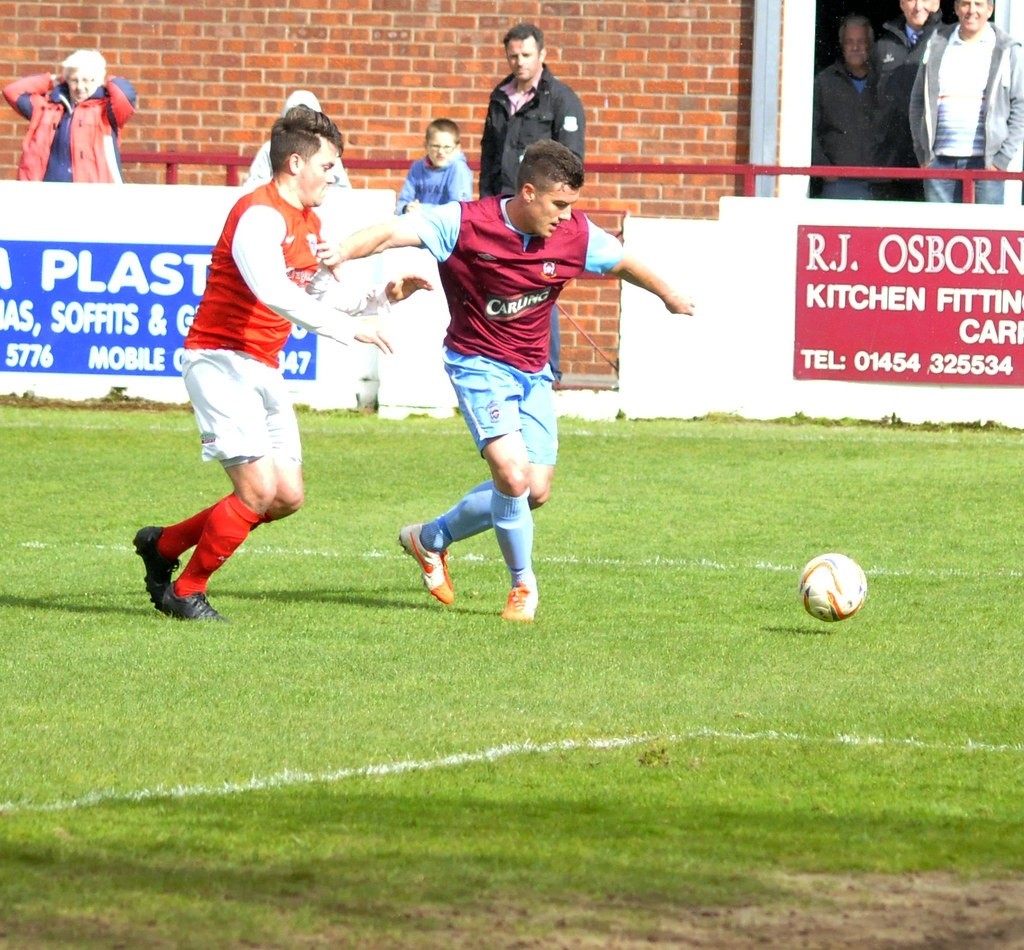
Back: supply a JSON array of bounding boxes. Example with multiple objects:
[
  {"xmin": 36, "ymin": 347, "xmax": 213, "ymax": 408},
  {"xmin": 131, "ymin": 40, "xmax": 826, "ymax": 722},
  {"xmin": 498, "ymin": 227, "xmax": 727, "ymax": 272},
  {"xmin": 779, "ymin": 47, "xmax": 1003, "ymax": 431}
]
[{"xmin": 426, "ymin": 142, "xmax": 457, "ymax": 154}]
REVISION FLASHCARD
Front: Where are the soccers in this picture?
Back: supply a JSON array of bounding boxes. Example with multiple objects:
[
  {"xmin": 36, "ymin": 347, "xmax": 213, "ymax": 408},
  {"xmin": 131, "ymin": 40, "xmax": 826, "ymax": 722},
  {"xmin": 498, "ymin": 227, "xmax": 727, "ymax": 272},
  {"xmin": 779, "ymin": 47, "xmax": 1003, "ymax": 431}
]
[{"xmin": 797, "ymin": 551, "xmax": 869, "ymax": 622}]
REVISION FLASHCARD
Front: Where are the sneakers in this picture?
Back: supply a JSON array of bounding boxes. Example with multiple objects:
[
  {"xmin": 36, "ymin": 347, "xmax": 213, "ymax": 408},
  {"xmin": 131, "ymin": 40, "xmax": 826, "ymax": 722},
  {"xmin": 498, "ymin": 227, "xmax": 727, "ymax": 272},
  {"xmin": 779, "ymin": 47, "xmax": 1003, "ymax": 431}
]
[
  {"xmin": 502, "ymin": 582, "xmax": 539, "ymax": 622},
  {"xmin": 133, "ymin": 526, "xmax": 180, "ymax": 611},
  {"xmin": 397, "ymin": 523, "xmax": 455, "ymax": 604},
  {"xmin": 161, "ymin": 581, "xmax": 227, "ymax": 623}
]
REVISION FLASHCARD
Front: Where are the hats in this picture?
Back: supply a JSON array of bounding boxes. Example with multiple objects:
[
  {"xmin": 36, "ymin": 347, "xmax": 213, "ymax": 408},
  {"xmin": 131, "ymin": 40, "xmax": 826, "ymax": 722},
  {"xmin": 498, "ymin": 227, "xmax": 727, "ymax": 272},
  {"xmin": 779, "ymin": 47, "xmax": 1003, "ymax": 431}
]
[{"xmin": 283, "ymin": 90, "xmax": 322, "ymax": 116}]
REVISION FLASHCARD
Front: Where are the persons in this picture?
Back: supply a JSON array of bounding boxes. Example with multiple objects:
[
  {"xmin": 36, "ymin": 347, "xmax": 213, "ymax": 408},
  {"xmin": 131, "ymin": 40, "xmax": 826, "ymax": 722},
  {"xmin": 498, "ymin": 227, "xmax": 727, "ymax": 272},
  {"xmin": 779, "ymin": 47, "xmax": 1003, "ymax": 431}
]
[
  {"xmin": 133, "ymin": 106, "xmax": 433, "ymax": 621},
  {"xmin": 399, "ymin": 118, "xmax": 472, "ymax": 215},
  {"xmin": 310, "ymin": 140, "xmax": 694, "ymax": 623},
  {"xmin": 2, "ymin": 50, "xmax": 138, "ymax": 182},
  {"xmin": 910, "ymin": 0, "xmax": 1024, "ymax": 204},
  {"xmin": 872, "ymin": 0, "xmax": 945, "ymax": 200},
  {"xmin": 246, "ymin": 90, "xmax": 351, "ymax": 186},
  {"xmin": 811, "ymin": 17, "xmax": 897, "ymax": 200},
  {"xmin": 479, "ymin": 23, "xmax": 585, "ymax": 385}
]
